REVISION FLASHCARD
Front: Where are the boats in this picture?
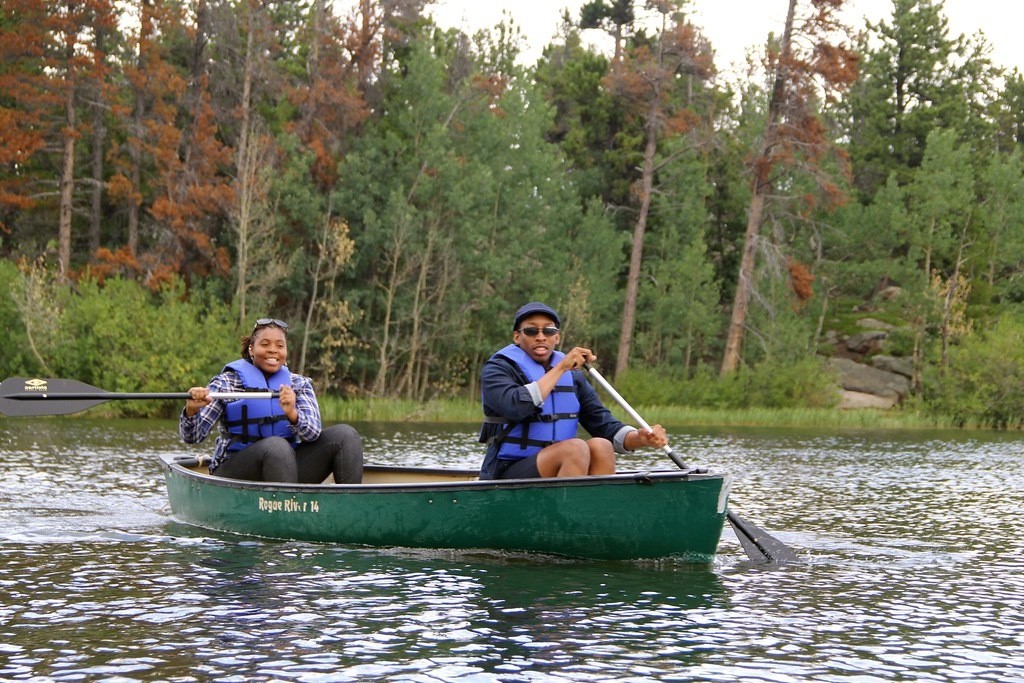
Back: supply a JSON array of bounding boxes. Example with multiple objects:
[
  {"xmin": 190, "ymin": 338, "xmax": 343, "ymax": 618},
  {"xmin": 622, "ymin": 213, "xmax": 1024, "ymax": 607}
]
[{"xmin": 158, "ymin": 451, "xmax": 735, "ymax": 566}]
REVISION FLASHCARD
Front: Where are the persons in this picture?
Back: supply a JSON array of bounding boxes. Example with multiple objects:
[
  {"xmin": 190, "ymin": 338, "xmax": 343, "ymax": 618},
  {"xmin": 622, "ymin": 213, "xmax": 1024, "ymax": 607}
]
[
  {"xmin": 178, "ymin": 319, "xmax": 364, "ymax": 485},
  {"xmin": 479, "ymin": 303, "xmax": 668, "ymax": 478}
]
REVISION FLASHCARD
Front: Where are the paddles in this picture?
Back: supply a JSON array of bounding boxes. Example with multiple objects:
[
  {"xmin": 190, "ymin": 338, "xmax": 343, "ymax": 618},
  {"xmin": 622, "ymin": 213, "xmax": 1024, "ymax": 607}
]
[
  {"xmin": 0, "ymin": 375, "xmax": 284, "ymax": 416},
  {"xmin": 581, "ymin": 355, "xmax": 798, "ymax": 566}
]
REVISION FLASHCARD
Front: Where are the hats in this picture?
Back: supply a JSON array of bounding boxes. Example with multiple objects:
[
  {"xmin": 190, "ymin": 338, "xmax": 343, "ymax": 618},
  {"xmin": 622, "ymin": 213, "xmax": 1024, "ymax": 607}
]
[{"xmin": 513, "ymin": 302, "xmax": 561, "ymax": 327}]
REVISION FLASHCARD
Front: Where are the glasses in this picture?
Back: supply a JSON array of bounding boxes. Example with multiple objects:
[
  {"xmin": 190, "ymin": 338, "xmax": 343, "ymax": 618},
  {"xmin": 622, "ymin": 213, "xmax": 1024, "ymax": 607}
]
[
  {"xmin": 517, "ymin": 325, "xmax": 558, "ymax": 335},
  {"xmin": 251, "ymin": 318, "xmax": 289, "ymax": 334}
]
